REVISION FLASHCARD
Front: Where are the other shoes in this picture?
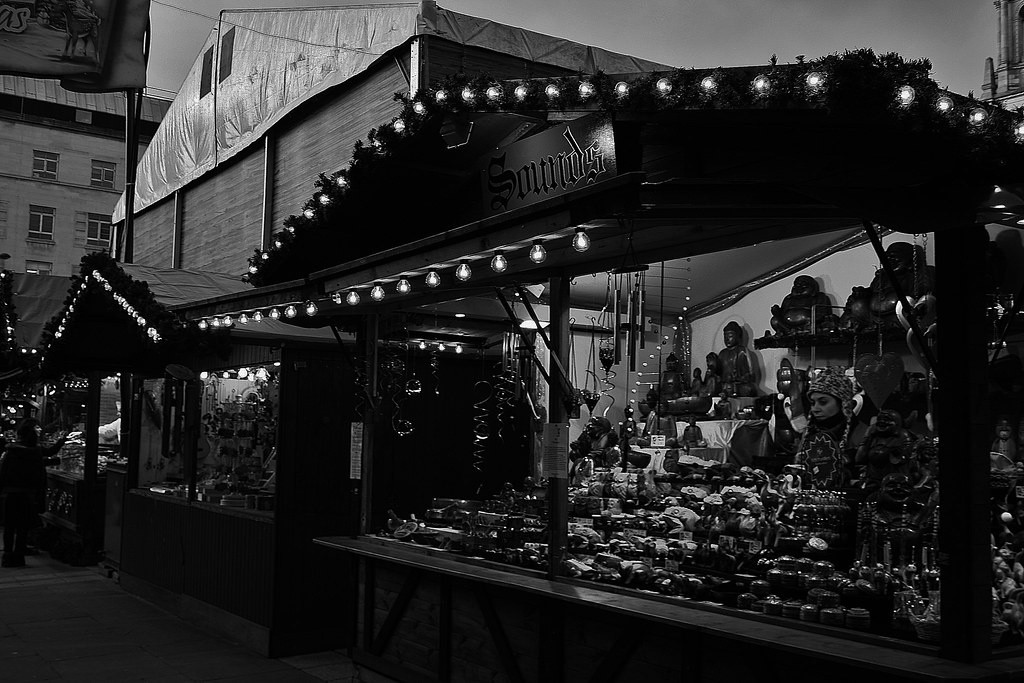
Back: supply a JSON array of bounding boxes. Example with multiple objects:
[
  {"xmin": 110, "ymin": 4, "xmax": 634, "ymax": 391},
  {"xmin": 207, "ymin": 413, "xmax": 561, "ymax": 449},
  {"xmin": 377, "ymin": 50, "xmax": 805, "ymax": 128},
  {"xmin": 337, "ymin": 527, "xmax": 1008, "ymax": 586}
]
[{"xmin": 2, "ymin": 552, "xmax": 25, "ymax": 567}]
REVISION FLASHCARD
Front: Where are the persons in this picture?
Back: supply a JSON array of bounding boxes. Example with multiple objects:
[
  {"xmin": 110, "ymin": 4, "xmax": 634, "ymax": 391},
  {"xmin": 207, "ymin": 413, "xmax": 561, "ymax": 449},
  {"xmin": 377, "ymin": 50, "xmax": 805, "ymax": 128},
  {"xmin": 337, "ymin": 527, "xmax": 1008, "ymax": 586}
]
[
  {"xmin": 847, "ymin": 242, "xmax": 929, "ymax": 333},
  {"xmin": 0, "ymin": 417, "xmax": 68, "ymax": 567},
  {"xmin": 570, "ymin": 417, "xmax": 618, "ymax": 471},
  {"xmin": 855, "ymin": 410, "xmax": 911, "ymax": 469},
  {"xmin": 771, "ymin": 275, "xmax": 832, "ymax": 335},
  {"xmin": 718, "ymin": 322, "xmax": 758, "ymax": 398},
  {"xmin": 620, "ymin": 406, "xmax": 637, "ymax": 444},
  {"xmin": 876, "ymin": 472, "xmax": 926, "ymax": 540},
  {"xmin": 638, "ymin": 398, "xmax": 677, "ymax": 448},
  {"xmin": 661, "ymin": 353, "xmax": 684, "ymax": 398},
  {"xmin": 715, "ymin": 391, "xmax": 731, "ymax": 419},
  {"xmin": 792, "ymin": 370, "xmax": 868, "ymax": 491},
  {"xmin": 680, "ymin": 416, "xmax": 703, "ymax": 447},
  {"xmin": 692, "ymin": 352, "xmax": 721, "ymax": 397},
  {"xmin": 69, "ymin": 401, "xmax": 121, "ymax": 444}
]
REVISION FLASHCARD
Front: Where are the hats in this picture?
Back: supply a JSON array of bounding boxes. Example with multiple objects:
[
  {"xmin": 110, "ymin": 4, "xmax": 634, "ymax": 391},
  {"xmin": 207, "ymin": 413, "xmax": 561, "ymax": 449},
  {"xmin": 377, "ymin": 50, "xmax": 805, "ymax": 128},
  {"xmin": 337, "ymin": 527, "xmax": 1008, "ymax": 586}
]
[{"xmin": 801, "ymin": 366, "xmax": 853, "ymax": 453}]
